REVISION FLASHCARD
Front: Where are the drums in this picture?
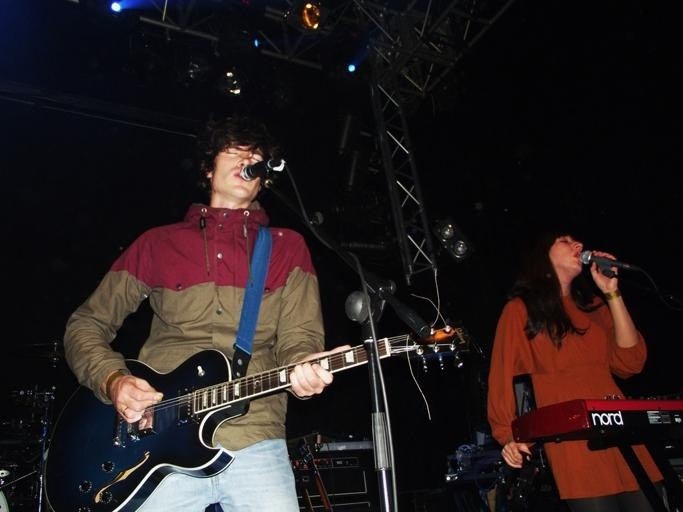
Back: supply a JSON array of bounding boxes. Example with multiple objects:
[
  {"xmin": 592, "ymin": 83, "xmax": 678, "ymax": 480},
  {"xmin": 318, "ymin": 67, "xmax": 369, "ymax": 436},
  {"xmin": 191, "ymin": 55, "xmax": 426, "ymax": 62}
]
[
  {"xmin": 0, "ymin": 389, "xmax": 51, "ymax": 445},
  {"xmin": 0, "ymin": 461, "xmax": 36, "ymax": 512},
  {"xmin": 17, "ymin": 322, "xmax": 66, "ymax": 366}
]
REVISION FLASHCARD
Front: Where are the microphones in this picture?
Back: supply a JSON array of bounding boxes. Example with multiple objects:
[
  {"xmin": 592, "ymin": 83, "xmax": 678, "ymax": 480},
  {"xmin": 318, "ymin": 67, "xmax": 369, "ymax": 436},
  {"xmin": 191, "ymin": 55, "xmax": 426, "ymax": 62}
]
[
  {"xmin": 579, "ymin": 250, "xmax": 630, "ymax": 269},
  {"xmin": 240, "ymin": 158, "xmax": 282, "ymax": 181}
]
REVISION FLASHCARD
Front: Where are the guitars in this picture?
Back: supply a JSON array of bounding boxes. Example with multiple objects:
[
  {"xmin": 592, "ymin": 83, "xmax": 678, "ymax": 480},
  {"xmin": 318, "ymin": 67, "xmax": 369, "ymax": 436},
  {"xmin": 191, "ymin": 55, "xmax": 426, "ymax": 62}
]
[{"xmin": 45, "ymin": 325, "xmax": 470, "ymax": 512}]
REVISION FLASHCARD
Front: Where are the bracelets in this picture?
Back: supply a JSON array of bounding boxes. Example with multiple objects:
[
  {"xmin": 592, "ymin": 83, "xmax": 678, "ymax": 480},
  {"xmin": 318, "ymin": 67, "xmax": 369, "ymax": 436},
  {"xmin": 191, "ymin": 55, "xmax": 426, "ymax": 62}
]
[{"xmin": 604, "ymin": 290, "xmax": 622, "ymax": 301}]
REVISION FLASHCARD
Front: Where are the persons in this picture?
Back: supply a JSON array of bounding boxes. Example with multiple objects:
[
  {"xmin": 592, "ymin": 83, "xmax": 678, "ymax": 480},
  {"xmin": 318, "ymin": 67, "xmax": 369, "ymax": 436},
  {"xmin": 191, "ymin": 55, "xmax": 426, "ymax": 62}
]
[
  {"xmin": 487, "ymin": 229, "xmax": 665, "ymax": 512},
  {"xmin": 64, "ymin": 113, "xmax": 352, "ymax": 512}
]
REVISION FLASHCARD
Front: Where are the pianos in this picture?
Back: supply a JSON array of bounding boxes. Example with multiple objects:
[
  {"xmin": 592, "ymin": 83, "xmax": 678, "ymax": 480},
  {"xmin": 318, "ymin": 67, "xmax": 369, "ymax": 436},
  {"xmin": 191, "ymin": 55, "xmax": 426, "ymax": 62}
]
[{"xmin": 510, "ymin": 393, "xmax": 682, "ymax": 443}]
